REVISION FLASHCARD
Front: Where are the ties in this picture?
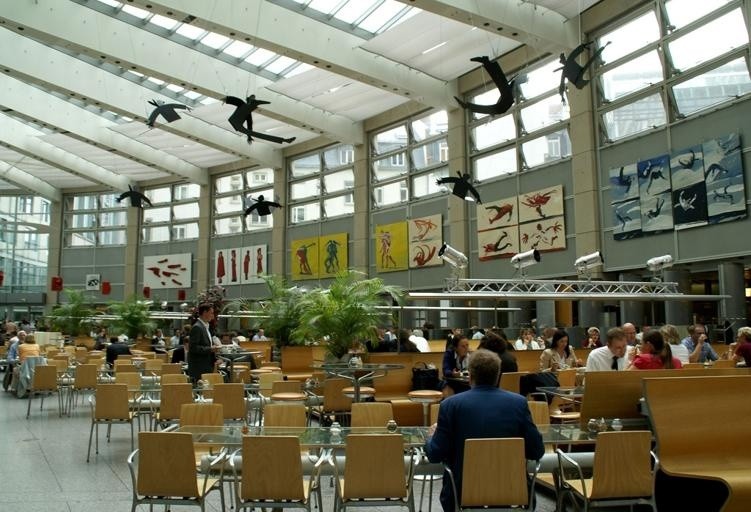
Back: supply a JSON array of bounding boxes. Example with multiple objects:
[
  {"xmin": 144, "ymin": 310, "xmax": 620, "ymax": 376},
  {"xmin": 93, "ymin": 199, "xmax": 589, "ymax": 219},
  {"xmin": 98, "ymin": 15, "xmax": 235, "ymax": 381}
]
[{"xmin": 612, "ymin": 356, "xmax": 618, "ymax": 371}]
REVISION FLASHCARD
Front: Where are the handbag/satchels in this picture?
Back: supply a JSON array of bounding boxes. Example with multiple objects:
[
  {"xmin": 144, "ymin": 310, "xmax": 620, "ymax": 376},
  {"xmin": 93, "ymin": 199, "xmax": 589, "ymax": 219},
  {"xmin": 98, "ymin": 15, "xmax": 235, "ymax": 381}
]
[{"xmin": 412, "ymin": 368, "xmax": 439, "ymax": 390}]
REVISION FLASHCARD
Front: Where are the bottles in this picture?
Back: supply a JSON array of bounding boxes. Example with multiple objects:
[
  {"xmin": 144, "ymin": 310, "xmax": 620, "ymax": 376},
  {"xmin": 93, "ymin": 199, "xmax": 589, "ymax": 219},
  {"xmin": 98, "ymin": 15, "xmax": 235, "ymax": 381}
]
[
  {"xmin": 386, "ymin": 419, "xmax": 399, "ymax": 433},
  {"xmin": 330, "ymin": 422, "xmax": 342, "ymax": 438},
  {"xmin": 587, "ymin": 418, "xmax": 623, "ymax": 433}
]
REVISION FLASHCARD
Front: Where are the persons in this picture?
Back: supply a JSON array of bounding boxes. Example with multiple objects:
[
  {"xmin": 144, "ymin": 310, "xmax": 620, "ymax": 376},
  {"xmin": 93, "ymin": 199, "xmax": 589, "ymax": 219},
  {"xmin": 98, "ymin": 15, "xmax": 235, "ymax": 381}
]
[
  {"xmin": 442, "ymin": 326, "xmax": 518, "ymax": 379},
  {"xmin": 518, "ymin": 326, "xmax": 584, "ymax": 371},
  {"xmin": 0, "ymin": 317, "xmax": 270, "ymax": 398},
  {"xmin": 350, "ymin": 326, "xmax": 430, "ymax": 354},
  {"xmin": 186, "ymin": 302, "xmax": 227, "ymax": 401},
  {"xmin": 580, "ymin": 322, "xmax": 751, "ymax": 372},
  {"xmin": 423, "ymin": 346, "xmax": 545, "ymax": 511}
]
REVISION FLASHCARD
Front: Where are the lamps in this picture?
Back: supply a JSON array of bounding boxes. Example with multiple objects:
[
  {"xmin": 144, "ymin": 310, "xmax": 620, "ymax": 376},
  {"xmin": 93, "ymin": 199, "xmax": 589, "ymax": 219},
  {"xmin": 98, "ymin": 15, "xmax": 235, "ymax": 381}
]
[
  {"xmin": 136, "ymin": 298, "xmax": 189, "ymax": 314},
  {"xmin": 435, "ymin": 243, "xmax": 685, "ymax": 297}
]
